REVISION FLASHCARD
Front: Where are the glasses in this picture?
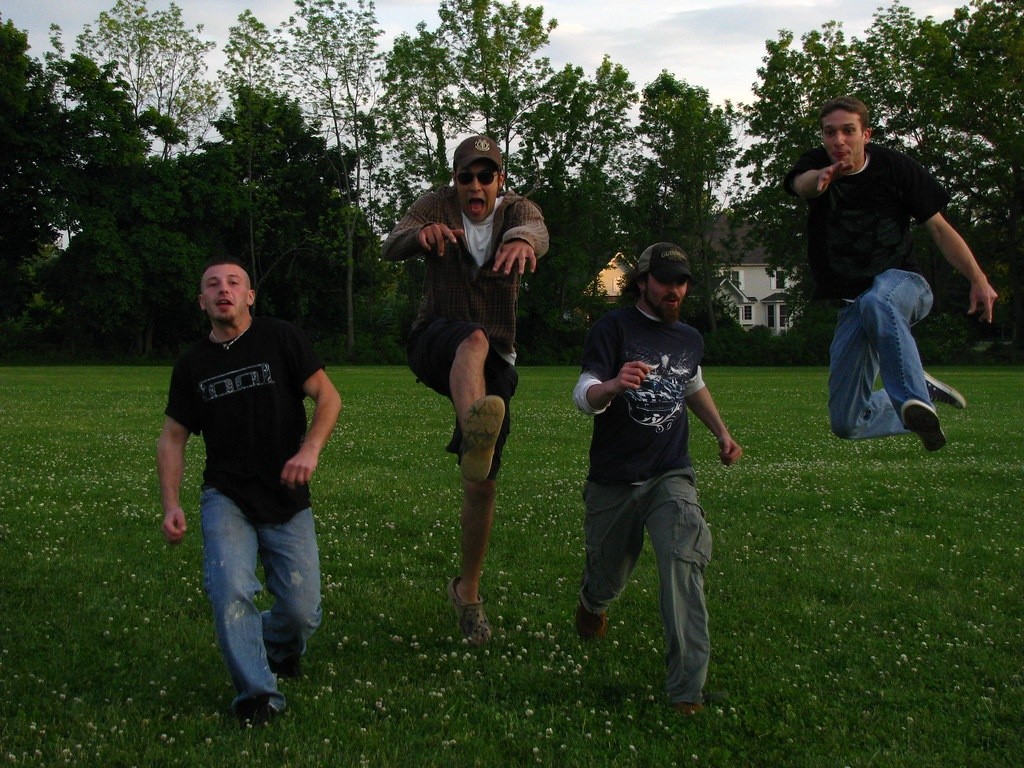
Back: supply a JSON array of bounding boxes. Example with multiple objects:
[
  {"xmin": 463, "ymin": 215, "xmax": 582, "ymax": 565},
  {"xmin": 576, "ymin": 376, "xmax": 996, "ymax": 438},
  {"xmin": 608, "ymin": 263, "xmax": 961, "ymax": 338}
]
[
  {"xmin": 455, "ymin": 169, "xmax": 498, "ymax": 186},
  {"xmin": 650, "ymin": 273, "xmax": 688, "ymax": 285}
]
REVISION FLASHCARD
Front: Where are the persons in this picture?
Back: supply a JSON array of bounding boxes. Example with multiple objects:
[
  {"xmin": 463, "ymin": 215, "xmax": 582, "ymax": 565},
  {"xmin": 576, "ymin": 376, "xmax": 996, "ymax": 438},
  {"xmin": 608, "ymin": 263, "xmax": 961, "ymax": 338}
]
[
  {"xmin": 787, "ymin": 95, "xmax": 998, "ymax": 450},
  {"xmin": 156, "ymin": 250, "xmax": 343, "ymax": 727},
  {"xmin": 379, "ymin": 136, "xmax": 552, "ymax": 645},
  {"xmin": 573, "ymin": 241, "xmax": 743, "ymax": 718}
]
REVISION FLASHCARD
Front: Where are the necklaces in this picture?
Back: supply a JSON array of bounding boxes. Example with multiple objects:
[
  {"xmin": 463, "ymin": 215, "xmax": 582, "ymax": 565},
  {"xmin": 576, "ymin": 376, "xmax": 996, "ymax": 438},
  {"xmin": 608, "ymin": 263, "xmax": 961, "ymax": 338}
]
[{"xmin": 210, "ymin": 316, "xmax": 253, "ymax": 351}]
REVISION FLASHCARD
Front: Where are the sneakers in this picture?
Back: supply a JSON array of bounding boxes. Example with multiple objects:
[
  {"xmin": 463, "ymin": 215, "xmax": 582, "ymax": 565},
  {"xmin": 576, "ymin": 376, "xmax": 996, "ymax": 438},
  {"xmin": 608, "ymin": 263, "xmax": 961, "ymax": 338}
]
[
  {"xmin": 901, "ymin": 399, "xmax": 946, "ymax": 451},
  {"xmin": 922, "ymin": 370, "xmax": 966, "ymax": 410}
]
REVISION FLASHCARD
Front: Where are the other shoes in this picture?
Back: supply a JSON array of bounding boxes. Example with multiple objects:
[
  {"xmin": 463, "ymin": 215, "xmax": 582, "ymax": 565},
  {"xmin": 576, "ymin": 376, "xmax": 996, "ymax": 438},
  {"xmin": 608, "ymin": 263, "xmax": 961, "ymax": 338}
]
[
  {"xmin": 237, "ymin": 694, "xmax": 274, "ymax": 728},
  {"xmin": 673, "ymin": 702, "xmax": 701, "ymax": 717},
  {"xmin": 281, "ymin": 656, "xmax": 302, "ymax": 678},
  {"xmin": 576, "ymin": 599, "xmax": 607, "ymax": 640}
]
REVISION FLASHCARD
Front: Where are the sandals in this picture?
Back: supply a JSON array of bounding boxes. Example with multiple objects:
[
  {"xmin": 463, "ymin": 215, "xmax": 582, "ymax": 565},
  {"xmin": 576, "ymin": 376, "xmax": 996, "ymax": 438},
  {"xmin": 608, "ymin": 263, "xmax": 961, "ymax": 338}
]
[
  {"xmin": 447, "ymin": 577, "xmax": 491, "ymax": 645},
  {"xmin": 460, "ymin": 395, "xmax": 506, "ymax": 482}
]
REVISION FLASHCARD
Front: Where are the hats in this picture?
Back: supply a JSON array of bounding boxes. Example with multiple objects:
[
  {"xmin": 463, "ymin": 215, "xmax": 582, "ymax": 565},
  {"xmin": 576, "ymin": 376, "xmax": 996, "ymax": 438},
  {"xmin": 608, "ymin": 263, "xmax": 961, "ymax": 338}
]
[
  {"xmin": 634, "ymin": 242, "xmax": 691, "ymax": 279},
  {"xmin": 453, "ymin": 136, "xmax": 502, "ymax": 171}
]
